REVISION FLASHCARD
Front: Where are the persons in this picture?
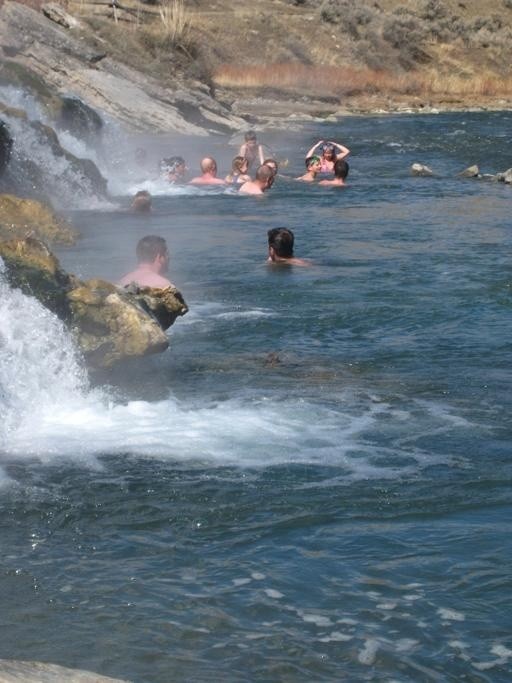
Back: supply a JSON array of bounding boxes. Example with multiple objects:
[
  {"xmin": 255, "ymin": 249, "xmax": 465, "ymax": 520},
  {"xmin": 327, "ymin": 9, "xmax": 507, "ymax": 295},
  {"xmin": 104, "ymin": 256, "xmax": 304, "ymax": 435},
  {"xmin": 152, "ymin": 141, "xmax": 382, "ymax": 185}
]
[
  {"xmin": 135, "ymin": 130, "xmax": 351, "ymax": 196},
  {"xmin": 121, "ymin": 234, "xmax": 176, "ymax": 289},
  {"xmin": 132, "ymin": 190, "xmax": 151, "ymax": 210},
  {"xmin": 265, "ymin": 226, "xmax": 312, "ymax": 266}
]
